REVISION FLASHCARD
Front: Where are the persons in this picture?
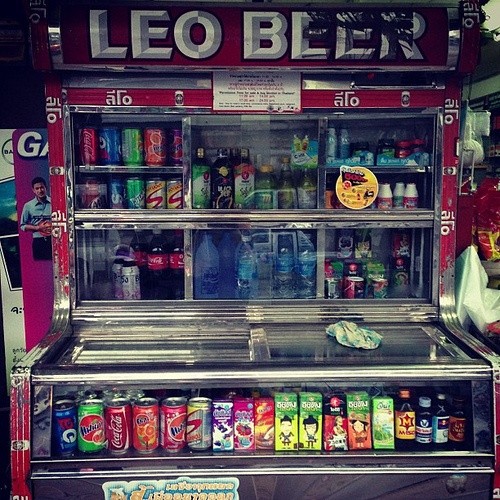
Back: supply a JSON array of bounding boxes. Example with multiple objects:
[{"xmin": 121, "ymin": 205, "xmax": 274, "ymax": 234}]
[{"xmin": 19, "ymin": 177, "xmax": 53, "ymax": 259}]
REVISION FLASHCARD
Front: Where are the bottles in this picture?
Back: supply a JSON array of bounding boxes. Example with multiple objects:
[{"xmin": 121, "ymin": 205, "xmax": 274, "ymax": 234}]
[
  {"xmin": 431, "ymin": 394, "xmax": 448, "ymax": 447},
  {"xmin": 415, "ymin": 396, "xmax": 433, "ymax": 445},
  {"xmin": 194, "ymin": 146, "xmax": 317, "ymax": 209},
  {"xmin": 394, "ymin": 390, "xmax": 415, "ymax": 449},
  {"xmin": 194, "ymin": 233, "xmax": 219, "ymax": 298},
  {"xmin": 369, "ymin": 180, "xmax": 418, "ymax": 210},
  {"xmin": 327, "ymin": 128, "xmax": 337, "ymax": 157},
  {"xmin": 297, "ymin": 234, "xmax": 316, "ymax": 299},
  {"xmin": 276, "ymin": 236, "xmax": 296, "ymax": 297},
  {"xmin": 338, "ymin": 129, "xmax": 349, "ymax": 158},
  {"xmin": 126, "ymin": 229, "xmax": 184, "ymax": 301},
  {"xmin": 234, "ymin": 235, "xmax": 257, "ymax": 298},
  {"xmin": 449, "ymin": 396, "xmax": 466, "ymax": 447}
]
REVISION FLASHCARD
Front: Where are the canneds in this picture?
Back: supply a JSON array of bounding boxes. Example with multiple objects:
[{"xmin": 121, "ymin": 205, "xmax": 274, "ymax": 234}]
[
  {"xmin": 325, "ymin": 276, "xmax": 388, "ymax": 299},
  {"xmin": 80, "ymin": 175, "xmax": 183, "ymax": 210},
  {"xmin": 76, "ymin": 126, "xmax": 182, "ymax": 167},
  {"xmin": 53, "ymin": 397, "xmax": 213, "ymax": 455},
  {"xmin": 348, "ymin": 138, "xmax": 426, "ymax": 159}
]
